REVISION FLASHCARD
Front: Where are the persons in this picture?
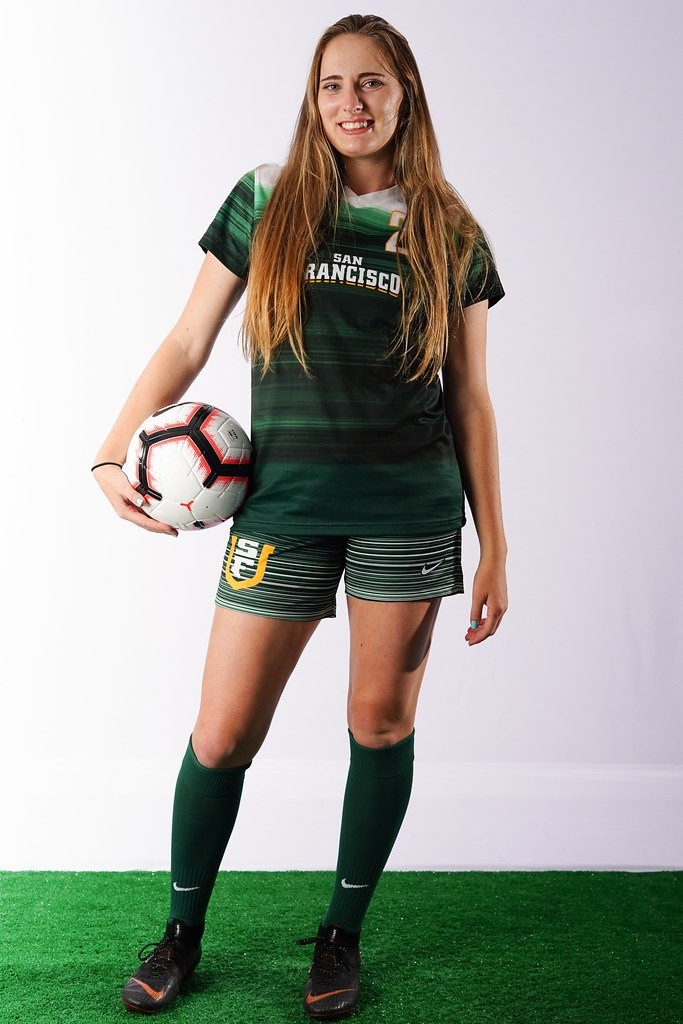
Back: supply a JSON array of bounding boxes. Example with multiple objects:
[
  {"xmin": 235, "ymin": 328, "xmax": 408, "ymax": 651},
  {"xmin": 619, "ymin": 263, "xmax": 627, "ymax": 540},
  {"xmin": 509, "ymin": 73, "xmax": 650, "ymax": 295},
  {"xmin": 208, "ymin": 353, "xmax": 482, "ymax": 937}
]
[{"xmin": 90, "ymin": 12, "xmax": 506, "ymax": 1021}]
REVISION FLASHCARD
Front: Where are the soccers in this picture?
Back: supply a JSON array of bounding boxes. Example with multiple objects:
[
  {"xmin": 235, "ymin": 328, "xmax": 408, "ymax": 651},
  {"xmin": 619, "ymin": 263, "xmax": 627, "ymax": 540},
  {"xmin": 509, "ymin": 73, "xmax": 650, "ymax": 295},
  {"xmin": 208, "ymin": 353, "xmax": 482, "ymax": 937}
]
[{"xmin": 126, "ymin": 402, "xmax": 249, "ymax": 529}]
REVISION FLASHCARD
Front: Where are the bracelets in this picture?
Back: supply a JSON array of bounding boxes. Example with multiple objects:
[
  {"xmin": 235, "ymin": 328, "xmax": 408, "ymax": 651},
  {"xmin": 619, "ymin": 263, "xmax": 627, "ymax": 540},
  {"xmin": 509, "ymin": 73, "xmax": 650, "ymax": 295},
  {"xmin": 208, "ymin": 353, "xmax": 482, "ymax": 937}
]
[{"xmin": 91, "ymin": 462, "xmax": 122, "ymax": 472}]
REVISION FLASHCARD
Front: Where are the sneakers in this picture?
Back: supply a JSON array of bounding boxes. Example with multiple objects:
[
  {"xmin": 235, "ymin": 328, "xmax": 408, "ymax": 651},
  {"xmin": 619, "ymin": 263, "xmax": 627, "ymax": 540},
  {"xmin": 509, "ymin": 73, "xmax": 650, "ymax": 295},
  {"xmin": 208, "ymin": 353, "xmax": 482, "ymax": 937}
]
[
  {"xmin": 121, "ymin": 917, "xmax": 203, "ymax": 1013},
  {"xmin": 304, "ymin": 922, "xmax": 361, "ymax": 1019}
]
